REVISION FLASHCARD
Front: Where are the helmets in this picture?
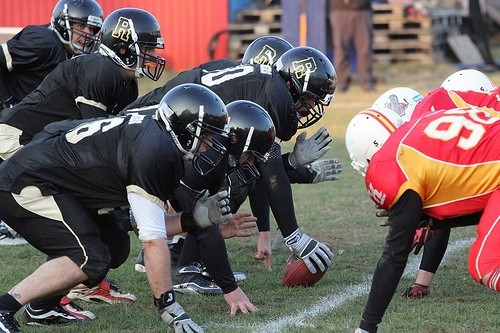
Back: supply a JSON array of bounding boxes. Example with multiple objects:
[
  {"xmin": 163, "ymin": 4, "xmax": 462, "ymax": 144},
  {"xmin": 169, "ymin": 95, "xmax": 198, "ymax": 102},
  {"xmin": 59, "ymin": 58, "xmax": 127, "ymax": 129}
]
[
  {"xmin": 225, "ymin": 101, "xmax": 276, "ymax": 188},
  {"xmin": 51, "ymin": 0, "xmax": 104, "ymax": 54},
  {"xmin": 102, "ymin": 8, "xmax": 166, "ymax": 82},
  {"xmin": 274, "ymin": 46, "xmax": 337, "ymax": 128},
  {"xmin": 345, "ymin": 106, "xmax": 405, "ymax": 178},
  {"xmin": 241, "ymin": 36, "xmax": 294, "ymax": 67},
  {"xmin": 159, "ymin": 83, "xmax": 229, "ymax": 175},
  {"xmin": 442, "ymin": 69, "xmax": 494, "ymax": 93},
  {"xmin": 372, "ymin": 87, "xmax": 423, "ymax": 123}
]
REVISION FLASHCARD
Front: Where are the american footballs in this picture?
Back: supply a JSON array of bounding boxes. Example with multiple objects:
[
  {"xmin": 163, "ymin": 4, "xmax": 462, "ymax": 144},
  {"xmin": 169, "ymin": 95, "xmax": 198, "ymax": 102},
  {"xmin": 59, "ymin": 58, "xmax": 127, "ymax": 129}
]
[{"xmin": 281, "ymin": 242, "xmax": 333, "ymax": 288}]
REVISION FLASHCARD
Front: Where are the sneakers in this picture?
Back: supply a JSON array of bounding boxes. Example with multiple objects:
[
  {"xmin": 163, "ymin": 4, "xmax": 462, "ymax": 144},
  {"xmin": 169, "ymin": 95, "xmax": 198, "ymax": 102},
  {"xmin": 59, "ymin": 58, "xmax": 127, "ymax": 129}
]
[
  {"xmin": 22, "ymin": 302, "xmax": 77, "ymax": 325},
  {"xmin": 0, "ymin": 310, "xmax": 24, "ymax": 333},
  {"xmin": 0, "ymin": 224, "xmax": 28, "ymax": 245},
  {"xmin": 68, "ymin": 278, "xmax": 137, "ymax": 304},
  {"xmin": 167, "ymin": 238, "xmax": 223, "ymax": 295},
  {"xmin": 135, "ymin": 247, "xmax": 146, "ymax": 272},
  {"xmin": 61, "ymin": 296, "xmax": 96, "ymax": 320}
]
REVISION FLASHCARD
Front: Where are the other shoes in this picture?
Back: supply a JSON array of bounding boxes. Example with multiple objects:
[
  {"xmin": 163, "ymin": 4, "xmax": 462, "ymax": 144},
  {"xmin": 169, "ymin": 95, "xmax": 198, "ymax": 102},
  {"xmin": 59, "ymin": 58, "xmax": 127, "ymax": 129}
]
[{"xmin": 232, "ymin": 272, "xmax": 246, "ymax": 282}]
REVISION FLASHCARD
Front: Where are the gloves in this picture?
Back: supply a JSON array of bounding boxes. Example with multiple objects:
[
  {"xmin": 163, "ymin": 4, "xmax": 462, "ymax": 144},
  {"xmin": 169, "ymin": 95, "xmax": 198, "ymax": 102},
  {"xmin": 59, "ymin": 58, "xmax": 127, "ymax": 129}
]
[
  {"xmin": 290, "ymin": 127, "xmax": 331, "ymax": 167},
  {"xmin": 401, "ymin": 284, "xmax": 430, "ymax": 298},
  {"xmin": 285, "ymin": 225, "xmax": 334, "ymax": 274},
  {"xmin": 409, "ymin": 226, "xmax": 429, "ymax": 255},
  {"xmin": 153, "ymin": 289, "xmax": 205, "ymax": 333},
  {"xmin": 308, "ymin": 159, "xmax": 341, "ymax": 182},
  {"xmin": 191, "ymin": 189, "xmax": 233, "ymax": 228}
]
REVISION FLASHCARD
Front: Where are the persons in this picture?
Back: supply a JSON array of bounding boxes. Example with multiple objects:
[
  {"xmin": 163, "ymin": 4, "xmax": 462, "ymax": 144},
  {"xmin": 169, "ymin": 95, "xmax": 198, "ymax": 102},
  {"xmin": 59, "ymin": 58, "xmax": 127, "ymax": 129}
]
[
  {"xmin": 0, "ymin": 0, "xmax": 500, "ymax": 333},
  {"xmin": 345, "ymin": 106, "xmax": 499, "ymax": 333}
]
